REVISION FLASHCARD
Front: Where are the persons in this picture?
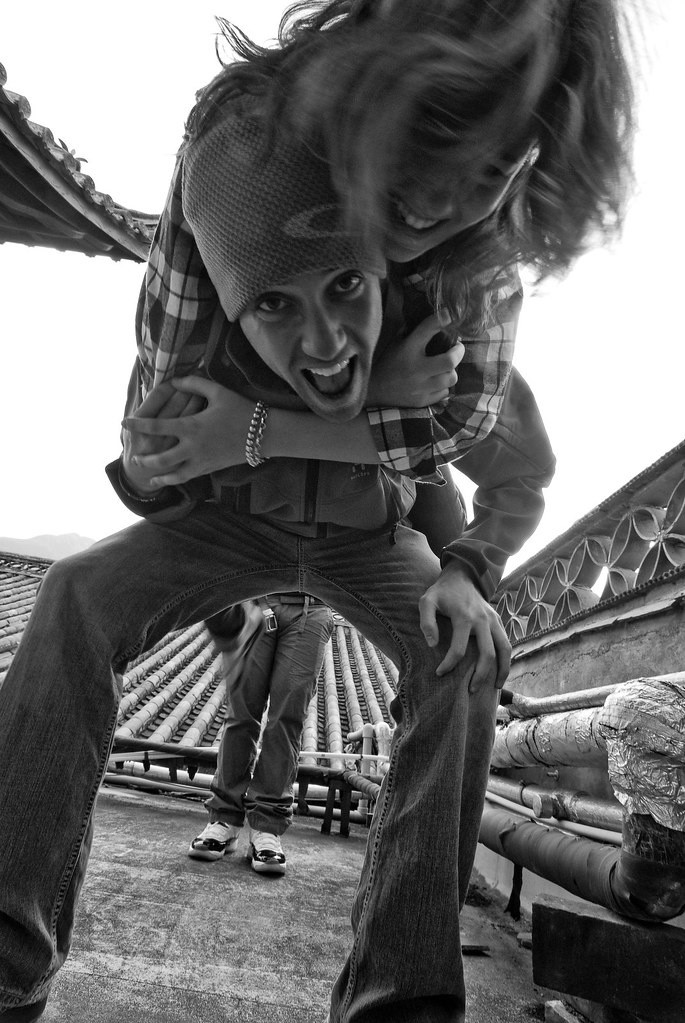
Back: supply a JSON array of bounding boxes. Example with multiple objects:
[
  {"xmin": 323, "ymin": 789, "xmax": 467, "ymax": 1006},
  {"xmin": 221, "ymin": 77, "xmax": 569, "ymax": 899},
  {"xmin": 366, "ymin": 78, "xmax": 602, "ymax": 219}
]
[
  {"xmin": 0, "ymin": 101, "xmax": 561, "ymax": 1022},
  {"xmin": 188, "ymin": 592, "xmax": 336, "ymax": 876},
  {"xmin": 117, "ymin": 0, "xmax": 638, "ymax": 681}
]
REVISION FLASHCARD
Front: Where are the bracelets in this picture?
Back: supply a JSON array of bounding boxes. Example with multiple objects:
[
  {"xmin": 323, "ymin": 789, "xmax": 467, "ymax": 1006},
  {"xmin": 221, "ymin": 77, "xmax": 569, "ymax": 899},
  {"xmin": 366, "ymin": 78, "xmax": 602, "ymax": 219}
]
[{"xmin": 244, "ymin": 401, "xmax": 270, "ymax": 468}]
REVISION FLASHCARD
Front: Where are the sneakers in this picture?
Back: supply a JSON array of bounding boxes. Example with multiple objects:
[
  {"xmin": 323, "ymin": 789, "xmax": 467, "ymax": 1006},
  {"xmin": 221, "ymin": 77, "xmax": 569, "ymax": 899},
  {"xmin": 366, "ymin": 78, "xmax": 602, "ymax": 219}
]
[
  {"xmin": 188, "ymin": 820, "xmax": 242, "ymax": 861},
  {"xmin": 246, "ymin": 826, "xmax": 287, "ymax": 874}
]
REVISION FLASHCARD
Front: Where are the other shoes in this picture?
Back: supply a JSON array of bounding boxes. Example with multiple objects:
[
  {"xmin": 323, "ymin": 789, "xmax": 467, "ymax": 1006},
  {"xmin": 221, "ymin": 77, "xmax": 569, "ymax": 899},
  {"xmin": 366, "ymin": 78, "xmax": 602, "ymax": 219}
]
[{"xmin": 216, "ymin": 601, "xmax": 271, "ymax": 694}]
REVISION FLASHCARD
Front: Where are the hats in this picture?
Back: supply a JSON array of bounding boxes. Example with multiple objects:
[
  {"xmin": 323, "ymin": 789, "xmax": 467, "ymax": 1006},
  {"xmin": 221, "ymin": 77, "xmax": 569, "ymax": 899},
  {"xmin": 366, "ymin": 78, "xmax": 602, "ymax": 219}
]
[{"xmin": 183, "ymin": 87, "xmax": 388, "ymax": 321}]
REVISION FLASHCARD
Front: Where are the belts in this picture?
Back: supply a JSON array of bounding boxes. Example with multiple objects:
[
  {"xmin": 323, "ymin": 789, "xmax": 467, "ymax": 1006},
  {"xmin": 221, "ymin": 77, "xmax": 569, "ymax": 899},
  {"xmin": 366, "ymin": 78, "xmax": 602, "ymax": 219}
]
[{"xmin": 257, "ymin": 595, "xmax": 325, "ymax": 634}]
